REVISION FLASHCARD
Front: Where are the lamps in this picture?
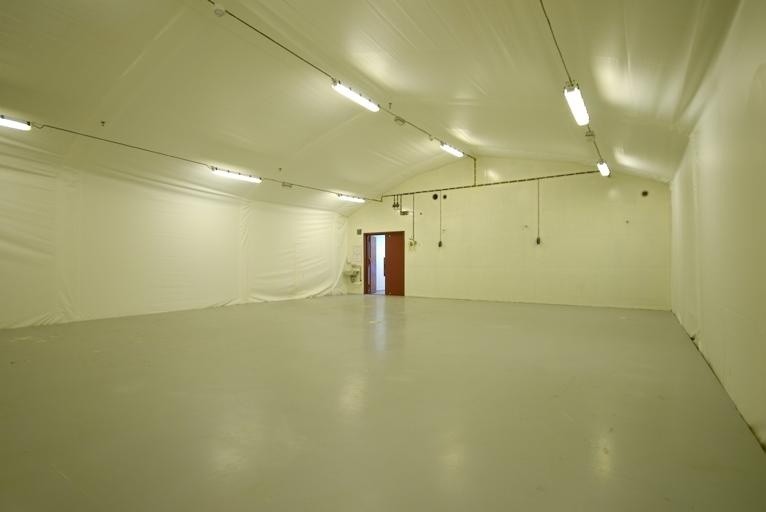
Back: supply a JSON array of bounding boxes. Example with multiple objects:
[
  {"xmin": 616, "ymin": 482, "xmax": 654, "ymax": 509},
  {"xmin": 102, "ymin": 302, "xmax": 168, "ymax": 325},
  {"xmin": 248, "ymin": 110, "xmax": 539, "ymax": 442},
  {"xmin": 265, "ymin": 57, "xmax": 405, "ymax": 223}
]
[
  {"xmin": 337, "ymin": 192, "xmax": 366, "ymax": 205},
  {"xmin": 210, "ymin": 165, "xmax": 263, "ymax": 185},
  {"xmin": 561, "ymin": 81, "xmax": 589, "ymax": 127},
  {"xmin": 597, "ymin": 159, "xmax": 611, "ymax": 178},
  {"xmin": 0, "ymin": 114, "xmax": 37, "ymax": 134},
  {"xmin": 439, "ymin": 143, "xmax": 465, "ymax": 159},
  {"xmin": 330, "ymin": 80, "xmax": 380, "ymax": 114}
]
